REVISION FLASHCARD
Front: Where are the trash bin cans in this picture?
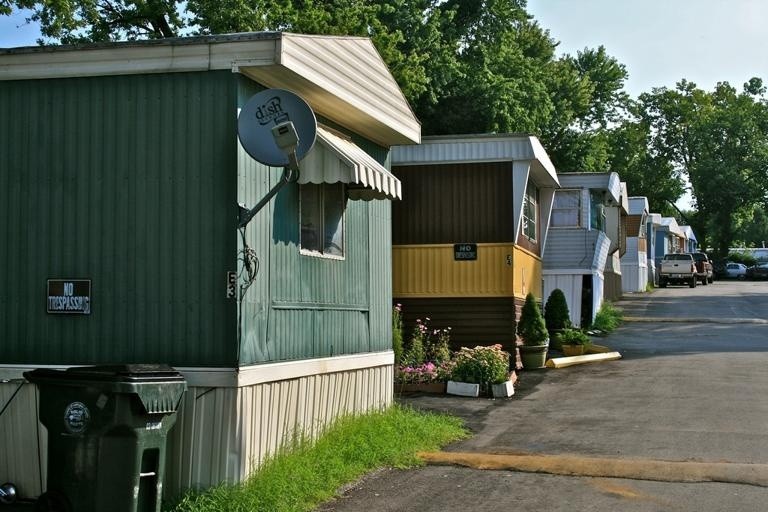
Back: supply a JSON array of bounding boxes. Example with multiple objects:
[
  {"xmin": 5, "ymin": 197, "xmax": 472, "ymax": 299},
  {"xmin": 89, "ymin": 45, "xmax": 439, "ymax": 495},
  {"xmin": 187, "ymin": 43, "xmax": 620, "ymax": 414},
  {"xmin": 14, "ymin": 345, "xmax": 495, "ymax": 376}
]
[{"xmin": 23, "ymin": 363, "xmax": 188, "ymax": 512}]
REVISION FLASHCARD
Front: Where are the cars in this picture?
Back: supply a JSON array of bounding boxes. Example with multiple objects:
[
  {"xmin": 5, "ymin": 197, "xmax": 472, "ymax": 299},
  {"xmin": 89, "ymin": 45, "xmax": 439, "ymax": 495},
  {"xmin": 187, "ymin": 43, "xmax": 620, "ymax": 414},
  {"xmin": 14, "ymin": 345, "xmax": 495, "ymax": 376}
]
[{"xmin": 725, "ymin": 262, "xmax": 768, "ymax": 281}]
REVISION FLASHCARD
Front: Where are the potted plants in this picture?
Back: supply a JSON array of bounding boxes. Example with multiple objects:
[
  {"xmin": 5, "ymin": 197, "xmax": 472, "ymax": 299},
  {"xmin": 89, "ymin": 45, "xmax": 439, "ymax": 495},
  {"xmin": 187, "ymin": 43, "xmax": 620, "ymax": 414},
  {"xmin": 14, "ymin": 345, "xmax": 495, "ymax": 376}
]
[
  {"xmin": 561, "ymin": 319, "xmax": 589, "ymax": 356},
  {"xmin": 518, "ymin": 293, "xmax": 550, "ymax": 369},
  {"xmin": 546, "ymin": 288, "xmax": 572, "ymax": 351}
]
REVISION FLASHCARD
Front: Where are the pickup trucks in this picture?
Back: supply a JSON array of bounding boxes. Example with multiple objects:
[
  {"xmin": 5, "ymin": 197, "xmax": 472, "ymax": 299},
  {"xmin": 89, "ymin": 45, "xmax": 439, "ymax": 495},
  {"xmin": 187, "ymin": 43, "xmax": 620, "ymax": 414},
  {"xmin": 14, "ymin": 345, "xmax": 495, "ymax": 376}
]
[{"xmin": 659, "ymin": 252, "xmax": 715, "ymax": 288}]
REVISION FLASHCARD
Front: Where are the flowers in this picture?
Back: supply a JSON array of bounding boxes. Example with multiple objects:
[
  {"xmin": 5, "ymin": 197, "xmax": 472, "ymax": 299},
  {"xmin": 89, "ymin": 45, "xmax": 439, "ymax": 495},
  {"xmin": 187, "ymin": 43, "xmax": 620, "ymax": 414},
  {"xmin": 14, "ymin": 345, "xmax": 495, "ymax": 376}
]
[{"xmin": 393, "ymin": 302, "xmax": 453, "ymax": 384}]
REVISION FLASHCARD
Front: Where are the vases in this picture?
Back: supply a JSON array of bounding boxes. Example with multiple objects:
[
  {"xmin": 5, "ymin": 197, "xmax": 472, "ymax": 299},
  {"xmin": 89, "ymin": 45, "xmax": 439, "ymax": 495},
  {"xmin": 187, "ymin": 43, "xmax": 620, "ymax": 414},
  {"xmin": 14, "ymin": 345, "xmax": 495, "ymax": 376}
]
[{"xmin": 394, "ymin": 384, "xmax": 445, "ymax": 393}]
